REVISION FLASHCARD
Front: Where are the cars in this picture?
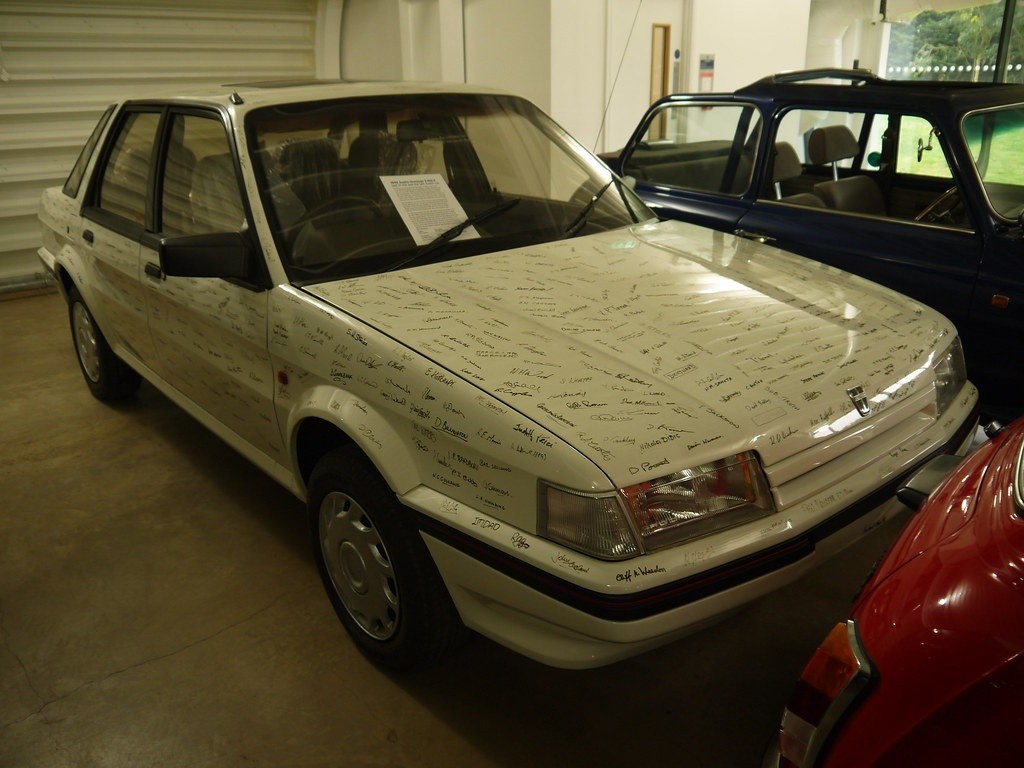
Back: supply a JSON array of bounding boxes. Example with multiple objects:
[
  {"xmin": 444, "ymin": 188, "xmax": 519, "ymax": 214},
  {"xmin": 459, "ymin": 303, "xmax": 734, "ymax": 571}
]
[
  {"xmin": 759, "ymin": 407, "xmax": 1023, "ymax": 768},
  {"xmin": 38, "ymin": 79, "xmax": 992, "ymax": 677},
  {"xmin": 593, "ymin": 56, "xmax": 1024, "ymax": 407}
]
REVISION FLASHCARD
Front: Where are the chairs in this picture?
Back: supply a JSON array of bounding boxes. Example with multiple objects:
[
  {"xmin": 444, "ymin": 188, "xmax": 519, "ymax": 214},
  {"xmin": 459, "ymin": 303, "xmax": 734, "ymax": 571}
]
[
  {"xmin": 808, "ymin": 125, "xmax": 886, "ymax": 214},
  {"xmin": 279, "ymin": 139, "xmax": 346, "ymax": 218},
  {"xmin": 740, "ymin": 142, "xmax": 829, "ymax": 208},
  {"xmin": 349, "ymin": 129, "xmax": 419, "ymax": 211},
  {"xmin": 188, "ymin": 151, "xmax": 306, "ymax": 241},
  {"xmin": 112, "ymin": 142, "xmax": 197, "ymax": 236}
]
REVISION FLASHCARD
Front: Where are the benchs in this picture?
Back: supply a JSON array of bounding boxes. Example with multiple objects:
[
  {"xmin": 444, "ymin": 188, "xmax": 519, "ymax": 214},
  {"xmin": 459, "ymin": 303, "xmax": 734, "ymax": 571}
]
[{"xmin": 624, "ymin": 138, "xmax": 748, "ymax": 192}]
